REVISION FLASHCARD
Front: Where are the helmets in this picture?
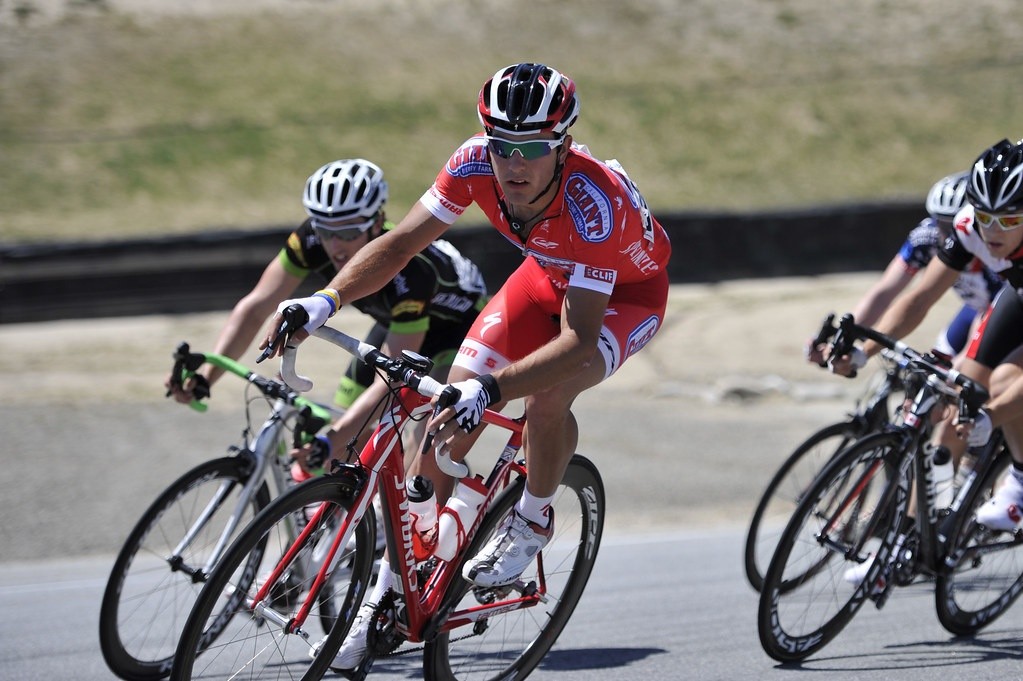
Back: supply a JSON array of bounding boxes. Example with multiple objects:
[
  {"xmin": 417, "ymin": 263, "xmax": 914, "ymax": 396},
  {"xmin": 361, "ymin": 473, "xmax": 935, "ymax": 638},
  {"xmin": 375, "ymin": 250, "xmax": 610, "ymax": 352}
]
[
  {"xmin": 478, "ymin": 63, "xmax": 580, "ymax": 137},
  {"xmin": 302, "ymin": 158, "xmax": 387, "ymax": 221},
  {"xmin": 926, "ymin": 171, "xmax": 970, "ymax": 222},
  {"xmin": 965, "ymin": 139, "xmax": 1023, "ymax": 218}
]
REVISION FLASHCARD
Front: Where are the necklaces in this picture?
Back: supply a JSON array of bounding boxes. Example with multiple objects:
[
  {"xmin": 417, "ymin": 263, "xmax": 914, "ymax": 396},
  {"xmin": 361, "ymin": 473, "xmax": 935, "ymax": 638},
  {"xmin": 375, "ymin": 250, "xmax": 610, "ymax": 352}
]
[{"xmin": 509, "ymin": 179, "xmax": 561, "ymax": 234}]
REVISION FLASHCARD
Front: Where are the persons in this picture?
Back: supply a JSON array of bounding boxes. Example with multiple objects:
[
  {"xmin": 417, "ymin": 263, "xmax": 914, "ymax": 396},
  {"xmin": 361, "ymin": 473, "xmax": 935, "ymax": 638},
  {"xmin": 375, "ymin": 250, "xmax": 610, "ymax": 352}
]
[
  {"xmin": 807, "ymin": 138, "xmax": 1023, "ymax": 585},
  {"xmin": 260, "ymin": 63, "xmax": 672, "ymax": 671},
  {"xmin": 165, "ymin": 158, "xmax": 488, "ymax": 617}
]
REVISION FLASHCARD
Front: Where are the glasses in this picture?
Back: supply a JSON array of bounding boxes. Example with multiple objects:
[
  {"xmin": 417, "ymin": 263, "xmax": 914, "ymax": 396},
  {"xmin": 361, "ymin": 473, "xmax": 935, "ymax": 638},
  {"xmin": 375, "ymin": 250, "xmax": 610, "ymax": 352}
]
[
  {"xmin": 483, "ymin": 133, "xmax": 568, "ymax": 160},
  {"xmin": 941, "ymin": 225, "xmax": 953, "ymax": 234},
  {"xmin": 975, "ymin": 209, "xmax": 1023, "ymax": 230},
  {"xmin": 311, "ymin": 219, "xmax": 377, "ymax": 240}
]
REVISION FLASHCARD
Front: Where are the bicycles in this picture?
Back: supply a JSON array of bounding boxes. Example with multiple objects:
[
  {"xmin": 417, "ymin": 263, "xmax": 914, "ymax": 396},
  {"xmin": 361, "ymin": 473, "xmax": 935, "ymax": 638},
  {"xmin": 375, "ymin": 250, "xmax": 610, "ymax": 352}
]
[
  {"xmin": 170, "ymin": 303, "xmax": 605, "ymax": 681},
  {"xmin": 98, "ymin": 342, "xmax": 388, "ymax": 681},
  {"xmin": 744, "ymin": 314, "xmax": 1005, "ymax": 597},
  {"xmin": 758, "ymin": 313, "xmax": 1023, "ymax": 663}
]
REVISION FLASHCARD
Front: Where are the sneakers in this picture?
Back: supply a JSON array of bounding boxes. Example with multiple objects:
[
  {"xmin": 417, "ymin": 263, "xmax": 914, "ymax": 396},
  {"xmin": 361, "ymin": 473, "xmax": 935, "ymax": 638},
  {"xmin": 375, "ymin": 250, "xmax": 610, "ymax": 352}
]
[
  {"xmin": 308, "ymin": 601, "xmax": 407, "ymax": 670},
  {"xmin": 345, "ymin": 496, "xmax": 386, "ymax": 550},
  {"xmin": 463, "ymin": 500, "xmax": 554, "ymax": 587}
]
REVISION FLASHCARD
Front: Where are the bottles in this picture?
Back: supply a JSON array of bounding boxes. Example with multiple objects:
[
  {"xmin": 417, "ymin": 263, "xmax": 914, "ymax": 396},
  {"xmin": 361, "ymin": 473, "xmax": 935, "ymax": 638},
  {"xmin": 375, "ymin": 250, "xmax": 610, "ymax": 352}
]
[
  {"xmin": 929, "ymin": 445, "xmax": 954, "ymax": 510},
  {"xmin": 432, "ymin": 474, "xmax": 489, "ymax": 564},
  {"xmin": 290, "ymin": 462, "xmax": 324, "ymax": 519},
  {"xmin": 405, "ymin": 474, "xmax": 439, "ymax": 565}
]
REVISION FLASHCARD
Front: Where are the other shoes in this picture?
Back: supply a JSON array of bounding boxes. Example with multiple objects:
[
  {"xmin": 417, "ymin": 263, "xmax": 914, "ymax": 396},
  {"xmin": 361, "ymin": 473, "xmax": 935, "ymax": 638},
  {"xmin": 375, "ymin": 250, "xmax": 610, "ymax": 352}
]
[
  {"xmin": 888, "ymin": 534, "xmax": 905, "ymax": 563},
  {"xmin": 977, "ymin": 484, "xmax": 1023, "ymax": 530}
]
